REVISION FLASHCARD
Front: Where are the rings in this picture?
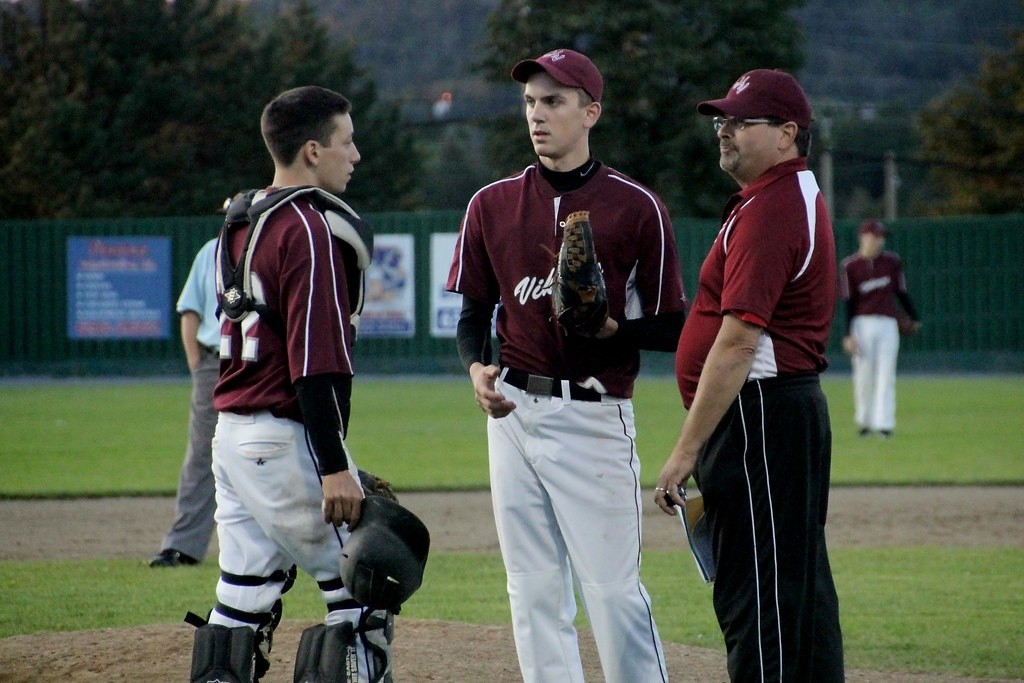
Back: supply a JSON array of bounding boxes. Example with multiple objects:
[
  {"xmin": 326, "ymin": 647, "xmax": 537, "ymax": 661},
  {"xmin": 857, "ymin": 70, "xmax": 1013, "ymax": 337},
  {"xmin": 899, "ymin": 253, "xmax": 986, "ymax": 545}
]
[{"xmin": 656, "ymin": 487, "xmax": 667, "ymax": 492}]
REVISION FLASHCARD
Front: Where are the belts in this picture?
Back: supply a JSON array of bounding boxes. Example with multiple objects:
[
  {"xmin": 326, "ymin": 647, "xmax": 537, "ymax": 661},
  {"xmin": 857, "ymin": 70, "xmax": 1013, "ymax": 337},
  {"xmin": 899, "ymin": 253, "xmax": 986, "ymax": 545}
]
[
  {"xmin": 205, "ymin": 348, "xmax": 220, "ymax": 359},
  {"xmin": 498, "ymin": 364, "xmax": 601, "ymax": 402}
]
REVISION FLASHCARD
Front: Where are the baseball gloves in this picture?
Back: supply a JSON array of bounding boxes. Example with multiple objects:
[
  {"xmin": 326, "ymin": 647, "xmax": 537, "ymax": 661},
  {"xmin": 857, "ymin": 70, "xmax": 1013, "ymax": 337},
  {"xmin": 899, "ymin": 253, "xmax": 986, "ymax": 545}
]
[
  {"xmin": 895, "ymin": 308, "xmax": 917, "ymax": 337},
  {"xmin": 539, "ymin": 209, "xmax": 610, "ymax": 339},
  {"xmin": 356, "ymin": 468, "xmax": 401, "ymax": 506}
]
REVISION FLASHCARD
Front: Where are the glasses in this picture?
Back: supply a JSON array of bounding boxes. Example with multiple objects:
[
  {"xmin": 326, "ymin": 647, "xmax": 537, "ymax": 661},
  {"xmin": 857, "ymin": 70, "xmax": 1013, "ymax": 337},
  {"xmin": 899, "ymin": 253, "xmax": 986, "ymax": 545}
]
[{"xmin": 712, "ymin": 116, "xmax": 788, "ymax": 131}]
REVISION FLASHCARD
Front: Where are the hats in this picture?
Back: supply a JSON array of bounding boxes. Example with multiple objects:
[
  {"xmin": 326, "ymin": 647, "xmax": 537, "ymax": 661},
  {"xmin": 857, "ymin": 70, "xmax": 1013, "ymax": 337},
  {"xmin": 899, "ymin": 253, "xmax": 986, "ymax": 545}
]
[
  {"xmin": 696, "ymin": 68, "xmax": 815, "ymax": 129},
  {"xmin": 859, "ymin": 219, "xmax": 892, "ymax": 236},
  {"xmin": 511, "ymin": 49, "xmax": 604, "ymax": 106}
]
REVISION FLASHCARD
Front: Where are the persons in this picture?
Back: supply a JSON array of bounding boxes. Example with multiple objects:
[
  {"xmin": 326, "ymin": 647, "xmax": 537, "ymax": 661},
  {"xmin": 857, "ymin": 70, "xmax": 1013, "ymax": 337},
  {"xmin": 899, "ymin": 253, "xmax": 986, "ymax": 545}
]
[
  {"xmin": 188, "ymin": 86, "xmax": 394, "ymax": 683},
  {"xmin": 655, "ymin": 69, "xmax": 844, "ymax": 683},
  {"xmin": 443, "ymin": 50, "xmax": 692, "ymax": 682},
  {"xmin": 149, "ymin": 193, "xmax": 237, "ymax": 568},
  {"xmin": 840, "ymin": 219, "xmax": 922, "ymax": 437}
]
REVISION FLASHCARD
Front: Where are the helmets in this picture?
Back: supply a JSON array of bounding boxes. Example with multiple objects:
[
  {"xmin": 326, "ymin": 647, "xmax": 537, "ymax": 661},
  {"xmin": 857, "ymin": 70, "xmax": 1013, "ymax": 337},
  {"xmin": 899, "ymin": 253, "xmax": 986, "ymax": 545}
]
[{"xmin": 338, "ymin": 495, "xmax": 430, "ymax": 610}]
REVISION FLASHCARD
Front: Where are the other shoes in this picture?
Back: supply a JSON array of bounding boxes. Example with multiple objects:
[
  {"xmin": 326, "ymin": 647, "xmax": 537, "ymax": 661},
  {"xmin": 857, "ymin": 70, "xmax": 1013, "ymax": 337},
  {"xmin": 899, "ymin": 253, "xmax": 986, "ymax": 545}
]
[{"xmin": 149, "ymin": 549, "xmax": 201, "ymax": 568}]
